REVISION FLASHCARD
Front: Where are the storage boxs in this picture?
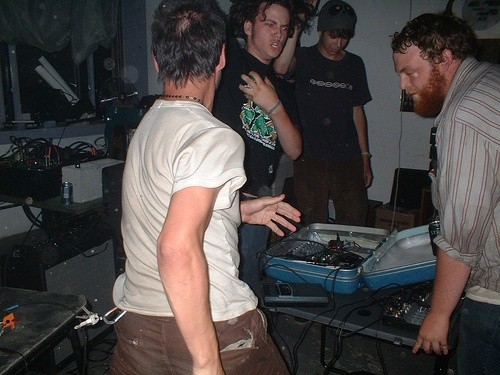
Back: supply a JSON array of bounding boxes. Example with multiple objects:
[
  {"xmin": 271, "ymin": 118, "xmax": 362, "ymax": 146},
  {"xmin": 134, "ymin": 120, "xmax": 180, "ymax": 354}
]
[
  {"xmin": 260, "ymin": 223, "xmax": 436, "ymax": 295},
  {"xmin": 60, "ymin": 157, "xmax": 126, "ymax": 203},
  {"xmin": 366, "ymin": 187, "xmax": 437, "ymax": 233}
]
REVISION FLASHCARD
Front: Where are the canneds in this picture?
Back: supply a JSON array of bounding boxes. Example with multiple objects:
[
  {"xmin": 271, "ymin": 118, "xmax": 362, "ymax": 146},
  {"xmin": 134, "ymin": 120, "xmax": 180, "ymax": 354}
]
[
  {"xmin": 60, "ymin": 181, "xmax": 73, "ymax": 207},
  {"xmin": 428, "ymin": 221, "xmax": 441, "ymax": 256}
]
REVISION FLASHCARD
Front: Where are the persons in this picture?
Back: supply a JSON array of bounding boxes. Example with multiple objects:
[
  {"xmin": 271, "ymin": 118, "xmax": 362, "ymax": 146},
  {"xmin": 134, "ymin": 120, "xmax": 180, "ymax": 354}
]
[
  {"xmin": 211, "ymin": 0, "xmax": 304, "ymax": 307},
  {"xmin": 112, "ymin": 0, "xmax": 302, "ymax": 375},
  {"xmin": 272, "ymin": 0, "xmax": 374, "ymax": 231},
  {"xmin": 391, "ymin": 12, "xmax": 500, "ymax": 374}
]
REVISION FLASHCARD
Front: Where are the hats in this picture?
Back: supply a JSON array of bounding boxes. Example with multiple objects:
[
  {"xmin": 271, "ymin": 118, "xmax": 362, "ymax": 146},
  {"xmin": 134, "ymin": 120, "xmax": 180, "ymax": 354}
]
[{"xmin": 317, "ymin": 0, "xmax": 357, "ymax": 32}]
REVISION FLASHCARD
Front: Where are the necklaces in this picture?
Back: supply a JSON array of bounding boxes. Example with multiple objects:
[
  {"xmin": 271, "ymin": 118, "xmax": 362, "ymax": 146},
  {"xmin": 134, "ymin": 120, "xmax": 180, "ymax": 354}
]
[{"xmin": 161, "ymin": 94, "xmax": 208, "ymax": 108}]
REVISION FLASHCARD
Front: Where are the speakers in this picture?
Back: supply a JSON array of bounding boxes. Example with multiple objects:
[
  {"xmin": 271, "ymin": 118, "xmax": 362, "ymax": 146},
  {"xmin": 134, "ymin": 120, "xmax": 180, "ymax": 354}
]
[{"xmin": 0, "ymin": 217, "xmax": 125, "ymax": 375}]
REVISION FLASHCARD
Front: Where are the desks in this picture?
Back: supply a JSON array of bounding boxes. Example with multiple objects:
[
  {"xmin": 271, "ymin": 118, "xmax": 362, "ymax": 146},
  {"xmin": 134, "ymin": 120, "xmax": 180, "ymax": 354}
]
[
  {"xmin": 240, "ymin": 266, "xmax": 466, "ymax": 375},
  {"xmin": 0, "ymin": 194, "xmax": 126, "ymax": 276},
  {"xmin": 0, "ymin": 286, "xmax": 93, "ymax": 375}
]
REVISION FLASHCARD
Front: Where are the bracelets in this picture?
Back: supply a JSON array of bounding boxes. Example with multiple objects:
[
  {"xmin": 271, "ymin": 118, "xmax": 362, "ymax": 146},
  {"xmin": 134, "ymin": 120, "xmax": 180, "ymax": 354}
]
[
  {"xmin": 269, "ymin": 101, "xmax": 280, "ymax": 114},
  {"xmin": 361, "ymin": 152, "xmax": 370, "ymax": 156}
]
